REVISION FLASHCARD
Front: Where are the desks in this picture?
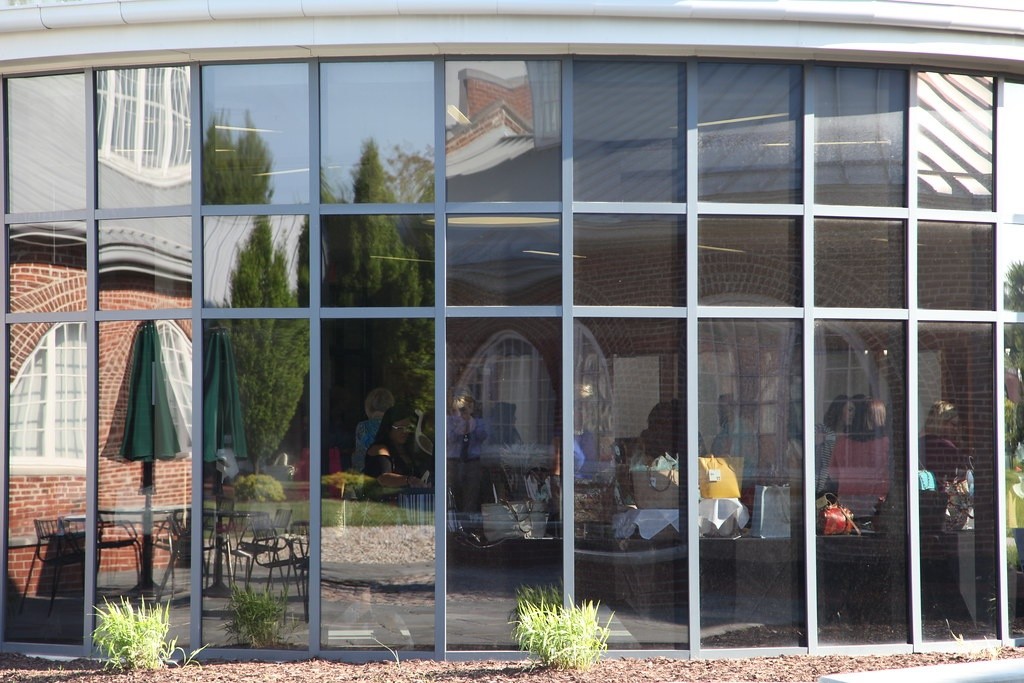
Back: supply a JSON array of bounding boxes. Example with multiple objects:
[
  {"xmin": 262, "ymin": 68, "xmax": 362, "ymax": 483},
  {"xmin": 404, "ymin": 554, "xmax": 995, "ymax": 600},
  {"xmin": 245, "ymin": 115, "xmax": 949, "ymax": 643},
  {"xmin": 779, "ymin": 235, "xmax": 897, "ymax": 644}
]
[
  {"xmin": 187, "ymin": 507, "xmax": 269, "ymax": 598},
  {"xmin": 97, "ymin": 504, "xmax": 191, "ymax": 597}
]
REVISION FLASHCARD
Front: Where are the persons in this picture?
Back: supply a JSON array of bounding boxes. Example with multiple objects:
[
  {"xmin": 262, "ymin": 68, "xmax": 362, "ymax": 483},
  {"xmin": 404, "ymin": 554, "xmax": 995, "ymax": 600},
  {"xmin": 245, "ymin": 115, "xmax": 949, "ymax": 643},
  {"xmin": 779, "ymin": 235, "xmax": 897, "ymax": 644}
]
[{"xmin": 291, "ymin": 385, "xmax": 1023, "ymax": 623}]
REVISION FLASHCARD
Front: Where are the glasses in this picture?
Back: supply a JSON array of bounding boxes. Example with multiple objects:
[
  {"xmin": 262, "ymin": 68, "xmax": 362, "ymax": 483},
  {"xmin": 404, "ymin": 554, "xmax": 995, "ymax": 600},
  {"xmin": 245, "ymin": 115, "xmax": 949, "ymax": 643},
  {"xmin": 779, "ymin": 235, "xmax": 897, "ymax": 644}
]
[{"xmin": 392, "ymin": 425, "xmax": 416, "ymax": 432}]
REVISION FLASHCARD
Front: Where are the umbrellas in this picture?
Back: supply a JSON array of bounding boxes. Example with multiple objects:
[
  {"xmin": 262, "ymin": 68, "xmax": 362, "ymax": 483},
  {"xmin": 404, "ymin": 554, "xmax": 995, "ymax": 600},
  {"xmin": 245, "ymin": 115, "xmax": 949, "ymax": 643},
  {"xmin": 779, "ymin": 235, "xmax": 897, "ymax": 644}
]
[
  {"xmin": 201, "ymin": 327, "xmax": 249, "ymax": 599},
  {"xmin": 120, "ymin": 321, "xmax": 181, "ymax": 597}
]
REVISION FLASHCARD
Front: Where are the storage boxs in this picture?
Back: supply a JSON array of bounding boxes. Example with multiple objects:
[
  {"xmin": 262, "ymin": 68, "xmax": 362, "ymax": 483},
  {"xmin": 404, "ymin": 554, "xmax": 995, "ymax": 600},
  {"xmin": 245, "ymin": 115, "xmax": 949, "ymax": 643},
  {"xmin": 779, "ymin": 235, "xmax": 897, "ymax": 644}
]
[{"xmin": 751, "ymin": 485, "xmax": 791, "ymax": 538}]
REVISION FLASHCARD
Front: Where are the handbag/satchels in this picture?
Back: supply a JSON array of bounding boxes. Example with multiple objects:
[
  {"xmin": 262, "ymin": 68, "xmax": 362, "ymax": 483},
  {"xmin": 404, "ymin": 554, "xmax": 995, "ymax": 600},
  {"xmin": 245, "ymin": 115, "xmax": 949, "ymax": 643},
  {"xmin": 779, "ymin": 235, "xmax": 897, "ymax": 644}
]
[
  {"xmin": 751, "ymin": 483, "xmax": 791, "ymax": 538},
  {"xmin": 574, "ymin": 483, "xmax": 617, "ymax": 524},
  {"xmin": 816, "ymin": 493, "xmax": 861, "ymax": 535},
  {"xmin": 633, "ymin": 469, "xmax": 680, "ymax": 510},
  {"xmin": 263, "ymin": 453, "xmax": 294, "ymax": 481},
  {"xmin": 481, "ymin": 499, "xmax": 533, "ymax": 541},
  {"xmin": 945, "ymin": 476, "xmax": 974, "ymax": 530},
  {"xmin": 699, "ymin": 453, "xmax": 744, "ymax": 499},
  {"xmin": 451, "ymin": 435, "xmax": 480, "ymax": 502}
]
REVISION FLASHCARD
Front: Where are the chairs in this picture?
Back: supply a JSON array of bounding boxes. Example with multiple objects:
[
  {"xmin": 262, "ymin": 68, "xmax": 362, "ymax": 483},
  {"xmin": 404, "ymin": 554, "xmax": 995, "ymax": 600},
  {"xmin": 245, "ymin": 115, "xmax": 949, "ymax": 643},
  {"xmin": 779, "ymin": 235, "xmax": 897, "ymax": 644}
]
[{"xmin": 20, "ymin": 498, "xmax": 310, "ymax": 624}]
